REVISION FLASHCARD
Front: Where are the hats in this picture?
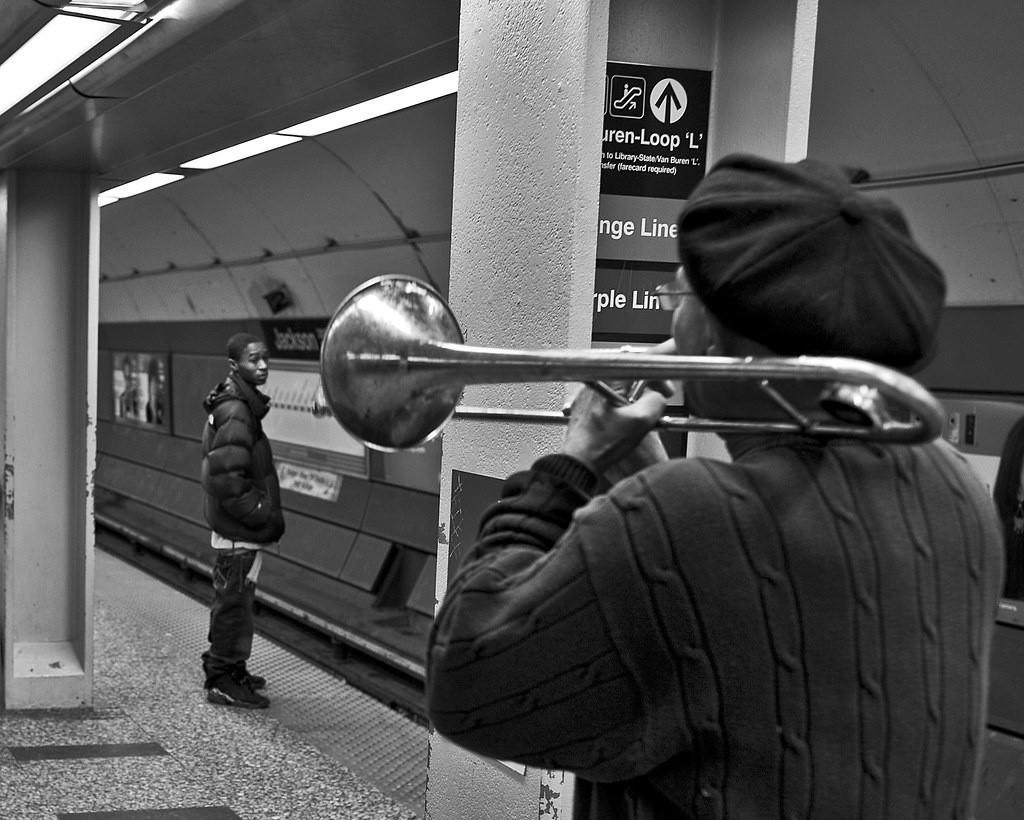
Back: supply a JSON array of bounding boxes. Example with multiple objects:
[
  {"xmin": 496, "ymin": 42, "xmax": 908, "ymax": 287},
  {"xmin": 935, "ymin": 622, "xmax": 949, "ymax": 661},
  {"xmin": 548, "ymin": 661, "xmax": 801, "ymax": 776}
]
[{"xmin": 676, "ymin": 151, "xmax": 947, "ymax": 376}]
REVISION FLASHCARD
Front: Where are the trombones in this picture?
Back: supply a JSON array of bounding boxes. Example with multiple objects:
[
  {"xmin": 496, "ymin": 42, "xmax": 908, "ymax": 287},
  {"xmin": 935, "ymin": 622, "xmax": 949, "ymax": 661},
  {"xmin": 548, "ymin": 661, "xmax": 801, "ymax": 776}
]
[{"xmin": 312, "ymin": 272, "xmax": 948, "ymax": 452}]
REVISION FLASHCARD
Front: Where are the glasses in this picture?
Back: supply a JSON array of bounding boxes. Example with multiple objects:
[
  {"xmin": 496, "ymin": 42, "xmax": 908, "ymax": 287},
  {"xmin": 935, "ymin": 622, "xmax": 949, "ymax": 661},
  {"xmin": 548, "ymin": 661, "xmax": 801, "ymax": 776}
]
[{"xmin": 650, "ymin": 281, "xmax": 700, "ymax": 311}]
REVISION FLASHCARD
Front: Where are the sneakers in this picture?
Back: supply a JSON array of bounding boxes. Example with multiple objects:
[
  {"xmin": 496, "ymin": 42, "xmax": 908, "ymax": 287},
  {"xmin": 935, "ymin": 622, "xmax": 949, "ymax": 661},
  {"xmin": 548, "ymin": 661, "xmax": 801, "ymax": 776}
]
[
  {"xmin": 208, "ymin": 677, "xmax": 271, "ymax": 707},
  {"xmin": 205, "ymin": 666, "xmax": 267, "ymax": 689}
]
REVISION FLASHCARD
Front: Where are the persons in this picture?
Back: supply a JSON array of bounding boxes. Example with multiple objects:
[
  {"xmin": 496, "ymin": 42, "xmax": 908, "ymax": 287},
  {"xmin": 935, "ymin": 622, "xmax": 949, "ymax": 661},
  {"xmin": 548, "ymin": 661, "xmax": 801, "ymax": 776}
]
[
  {"xmin": 427, "ymin": 153, "xmax": 1004, "ymax": 820},
  {"xmin": 201, "ymin": 333, "xmax": 287, "ymax": 710}
]
